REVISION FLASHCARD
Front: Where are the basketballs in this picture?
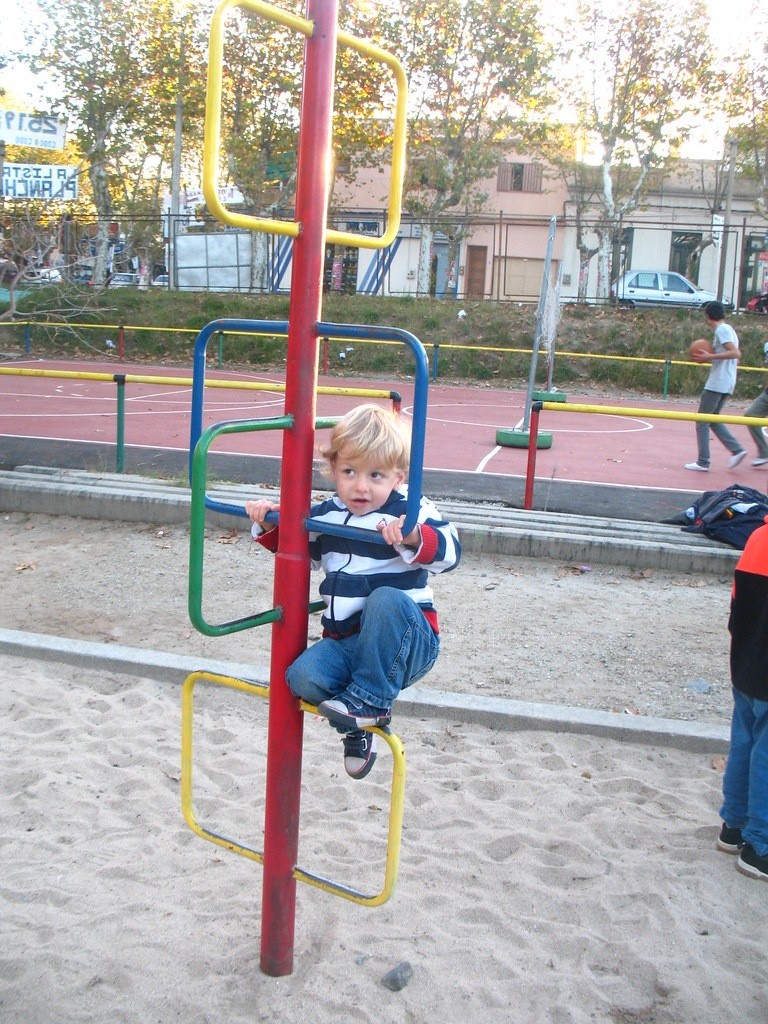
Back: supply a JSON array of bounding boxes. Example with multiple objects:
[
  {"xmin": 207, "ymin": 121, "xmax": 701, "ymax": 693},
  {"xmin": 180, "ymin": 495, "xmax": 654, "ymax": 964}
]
[{"xmin": 689, "ymin": 339, "xmax": 713, "ymax": 354}]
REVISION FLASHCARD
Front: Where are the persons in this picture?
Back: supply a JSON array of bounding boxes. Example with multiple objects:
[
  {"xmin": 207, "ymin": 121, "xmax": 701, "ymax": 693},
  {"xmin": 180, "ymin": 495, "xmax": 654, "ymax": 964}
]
[
  {"xmin": 717, "ymin": 516, "xmax": 768, "ymax": 882},
  {"xmin": 684, "ymin": 301, "xmax": 747, "ymax": 471},
  {"xmin": 744, "ymin": 340, "xmax": 768, "ymax": 467},
  {"xmin": 245, "ymin": 405, "xmax": 462, "ymax": 779}
]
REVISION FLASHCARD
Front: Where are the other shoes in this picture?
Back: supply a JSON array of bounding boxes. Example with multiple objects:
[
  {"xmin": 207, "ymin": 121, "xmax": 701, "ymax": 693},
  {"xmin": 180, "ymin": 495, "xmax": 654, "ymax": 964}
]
[
  {"xmin": 684, "ymin": 462, "xmax": 708, "ymax": 471},
  {"xmin": 736, "ymin": 844, "xmax": 768, "ymax": 882},
  {"xmin": 718, "ymin": 822, "xmax": 746, "ymax": 851},
  {"xmin": 751, "ymin": 457, "xmax": 768, "ymax": 466},
  {"xmin": 728, "ymin": 451, "xmax": 746, "ymax": 468}
]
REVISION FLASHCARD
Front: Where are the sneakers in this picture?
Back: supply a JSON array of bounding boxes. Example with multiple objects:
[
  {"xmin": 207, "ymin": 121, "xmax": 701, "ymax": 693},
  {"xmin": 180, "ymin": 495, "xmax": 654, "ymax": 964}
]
[
  {"xmin": 341, "ymin": 730, "xmax": 377, "ymax": 779},
  {"xmin": 317, "ymin": 690, "xmax": 391, "ymax": 728}
]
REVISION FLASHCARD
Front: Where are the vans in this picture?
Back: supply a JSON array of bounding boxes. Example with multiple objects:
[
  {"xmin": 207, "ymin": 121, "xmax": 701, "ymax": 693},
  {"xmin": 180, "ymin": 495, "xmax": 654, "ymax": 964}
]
[{"xmin": 107, "ymin": 272, "xmax": 145, "ymax": 290}]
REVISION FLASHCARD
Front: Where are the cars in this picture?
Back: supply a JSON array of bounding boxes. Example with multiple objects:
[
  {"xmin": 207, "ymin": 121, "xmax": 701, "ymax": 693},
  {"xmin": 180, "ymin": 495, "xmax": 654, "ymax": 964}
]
[
  {"xmin": 608, "ymin": 270, "xmax": 736, "ymax": 318},
  {"xmin": 153, "ymin": 275, "xmax": 169, "ymax": 291},
  {"xmin": 748, "ymin": 293, "xmax": 768, "ymax": 314},
  {"xmin": 0, "ymin": 258, "xmax": 103, "ymax": 304}
]
